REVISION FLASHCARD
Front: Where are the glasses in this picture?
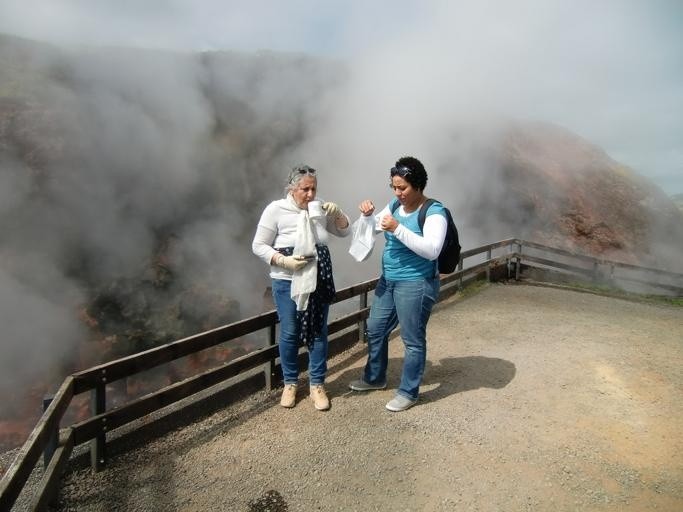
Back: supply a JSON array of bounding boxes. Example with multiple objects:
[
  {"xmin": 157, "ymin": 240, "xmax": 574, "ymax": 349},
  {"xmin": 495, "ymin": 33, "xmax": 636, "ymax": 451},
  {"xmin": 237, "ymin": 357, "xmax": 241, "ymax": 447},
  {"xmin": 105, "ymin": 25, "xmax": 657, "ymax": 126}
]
[
  {"xmin": 289, "ymin": 169, "xmax": 316, "ymax": 184},
  {"xmin": 391, "ymin": 167, "xmax": 413, "ymax": 181}
]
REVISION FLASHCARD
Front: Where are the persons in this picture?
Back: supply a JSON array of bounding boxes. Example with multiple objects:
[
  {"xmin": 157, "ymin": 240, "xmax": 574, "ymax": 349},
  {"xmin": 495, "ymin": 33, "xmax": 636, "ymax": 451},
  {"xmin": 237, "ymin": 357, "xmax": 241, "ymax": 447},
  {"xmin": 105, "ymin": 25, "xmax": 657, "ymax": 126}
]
[
  {"xmin": 251, "ymin": 164, "xmax": 351, "ymax": 411},
  {"xmin": 351, "ymin": 156, "xmax": 449, "ymax": 413}
]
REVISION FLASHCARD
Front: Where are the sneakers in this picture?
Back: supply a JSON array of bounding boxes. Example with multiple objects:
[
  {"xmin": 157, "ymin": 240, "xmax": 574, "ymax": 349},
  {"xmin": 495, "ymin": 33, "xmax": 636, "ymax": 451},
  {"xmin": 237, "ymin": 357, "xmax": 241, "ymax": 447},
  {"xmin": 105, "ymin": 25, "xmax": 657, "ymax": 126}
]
[
  {"xmin": 310, "ymin": 384, "xmax": 329, "ymax": 411},
  {"xmin": 281, "ymin": 383, "xmax": 299, "ymax": 407},
  {"xmin": 385, "ymin": 394, "xmax": 417, "ymax": 412},
  {"xmin": 348, "ymin": 379, "xmax": 387, "ymax": 391}
]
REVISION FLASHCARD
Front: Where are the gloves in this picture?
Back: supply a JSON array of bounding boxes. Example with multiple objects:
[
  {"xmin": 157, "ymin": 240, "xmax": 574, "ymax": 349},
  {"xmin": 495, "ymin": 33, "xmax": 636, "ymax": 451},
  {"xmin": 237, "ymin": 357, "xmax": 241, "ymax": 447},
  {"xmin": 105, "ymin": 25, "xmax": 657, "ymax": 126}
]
[
  {"xmin": 323, "ymin": 202, "xmax": 342, "ymax": 218},
  {"xmin": 276, "ymin": 254, "xmax": 308, "ymax": 271}
]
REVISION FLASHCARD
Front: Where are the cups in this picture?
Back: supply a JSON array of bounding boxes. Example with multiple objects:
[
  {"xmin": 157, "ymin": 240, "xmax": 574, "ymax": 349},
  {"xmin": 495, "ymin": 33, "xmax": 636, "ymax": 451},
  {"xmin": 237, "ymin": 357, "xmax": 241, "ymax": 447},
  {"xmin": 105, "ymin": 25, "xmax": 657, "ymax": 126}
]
[
  {"xmin": 308, "ymin": 200, "xmax": 328, "ymax": 220},
  {"xmin": 375, "ymin": 214, "xmax": 391, "ymax": 232}
]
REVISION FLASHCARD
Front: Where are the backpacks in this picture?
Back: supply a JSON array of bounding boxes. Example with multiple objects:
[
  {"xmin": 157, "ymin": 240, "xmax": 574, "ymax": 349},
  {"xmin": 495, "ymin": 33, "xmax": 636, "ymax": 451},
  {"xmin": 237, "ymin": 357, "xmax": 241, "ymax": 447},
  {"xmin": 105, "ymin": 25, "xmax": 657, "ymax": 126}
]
[{"xmin": 392, "ymin": 198, "xmax": 461, "ymax": 274}]
